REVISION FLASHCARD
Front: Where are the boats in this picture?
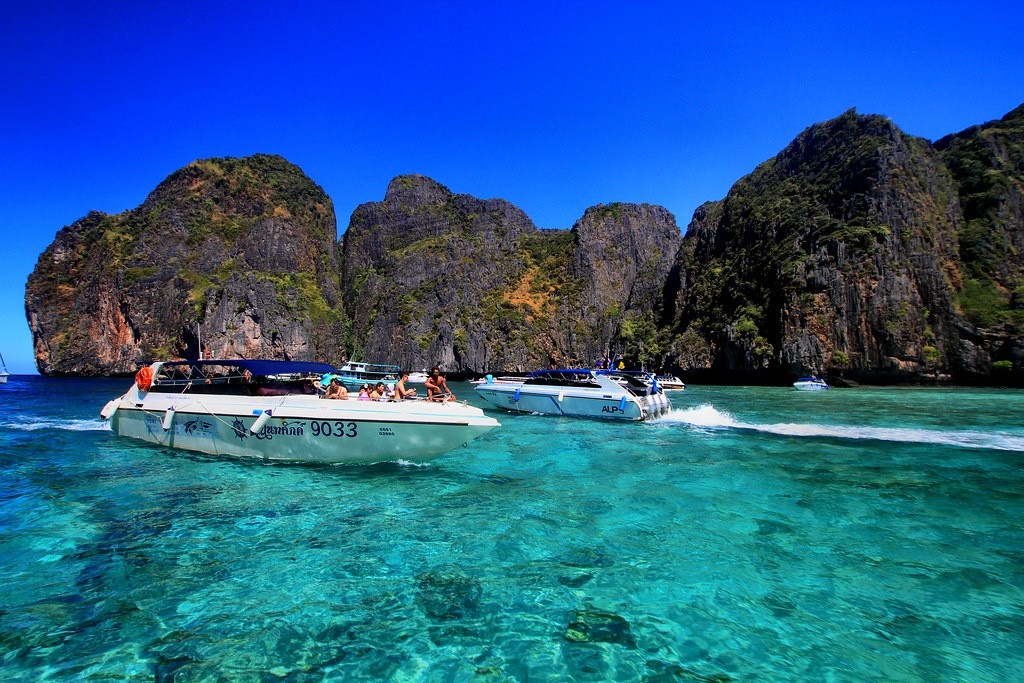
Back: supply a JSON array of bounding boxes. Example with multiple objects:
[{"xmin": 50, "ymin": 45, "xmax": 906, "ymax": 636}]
[
  {"xmin": 406, "ymin": 372, "xmax": 428, "ymax": 383},
  {"xmin": 110, "ymin": 361, "xmax": 503, "ymax": 467},
  {"xmin": 317, "ymin": 360, "xmax": 401, "ymax": 391},
  {"xmin": 793, "ymin": 375, "xmax": 828, "ymax": 390},
  {"xmin": 474, "ymin": 368, "xmax": 667, "ymax": 423},
  {"xmin": 648, "ymin": 373, "xmax": 685, "ymax": 389}
]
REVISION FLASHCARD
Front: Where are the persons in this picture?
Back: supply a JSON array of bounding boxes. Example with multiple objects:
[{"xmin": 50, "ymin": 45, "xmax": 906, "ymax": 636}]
[
  {"xmin": 357, "ymin": 372, "xmax": 417, "ymax": 402},
  {"xmin": 550, "ymin": 350, "xmax": 557, "ymax": 370},
  {"xmin": 653, "ymin": 372, "xmax": 673, "ymax": 380},
  {"xmin": 325, "ymin": 378, "xmax": 348, "ymax": 400},
  {"xmin": 424, "ymin": 366, "xmax": 456, "ymax": 402},
  {"xmin": 245, "ymin": 369, "xmax": 251, "ymax": 381}
]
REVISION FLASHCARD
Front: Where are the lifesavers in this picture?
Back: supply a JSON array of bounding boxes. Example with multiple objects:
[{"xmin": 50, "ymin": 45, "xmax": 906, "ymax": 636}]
[{"xmin": 137, "ymin": 367, "xmax": 152, "ymax": 391}]
